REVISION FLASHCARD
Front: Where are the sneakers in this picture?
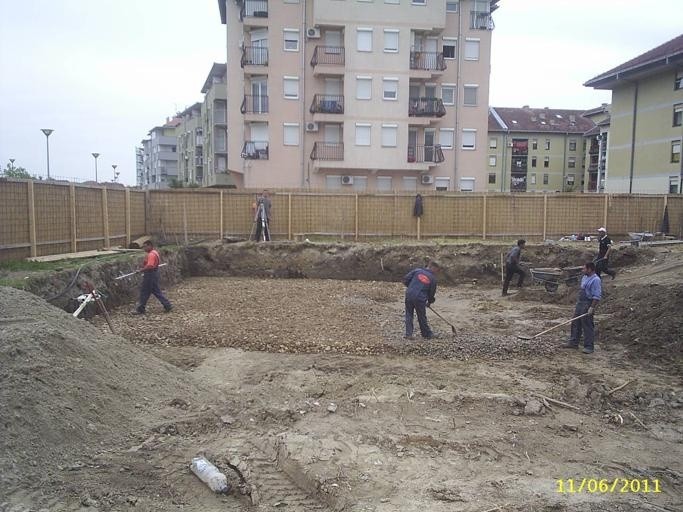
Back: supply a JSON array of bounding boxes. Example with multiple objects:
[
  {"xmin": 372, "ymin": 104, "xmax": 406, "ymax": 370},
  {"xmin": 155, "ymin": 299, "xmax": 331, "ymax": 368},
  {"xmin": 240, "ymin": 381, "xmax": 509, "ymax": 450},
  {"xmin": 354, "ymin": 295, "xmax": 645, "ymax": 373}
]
[
  {"xmin": 583, "ymin": 346, "xmax": 593, "ymax": 354},
  {"xmin": 163, "ymin": 307, "xmax": 173, "ymax": 313},
  {"xmin": 562, "ymin": 343, "xmax": 579, "ymax": 350},
  {"xmin": 612, "ymin": 271, "xmax": 616, "ymax": 280},
  {"xmin": 130, "ymin": 309, "xmax": 147, "ymax": 314}
]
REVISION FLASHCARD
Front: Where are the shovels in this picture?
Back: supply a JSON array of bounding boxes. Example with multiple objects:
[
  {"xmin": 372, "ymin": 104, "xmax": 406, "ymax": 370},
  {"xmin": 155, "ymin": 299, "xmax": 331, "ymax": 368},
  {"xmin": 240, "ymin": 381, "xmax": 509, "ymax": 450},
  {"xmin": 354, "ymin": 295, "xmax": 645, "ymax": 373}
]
[
  {"xmin": 426, "ymin": 303, "xmax": 458, "ymax": 338},
  {"xmin": 517, "ymin": 313, "xmax": 589, "ymax": 340},
  {"xmin": 500, "ymin": 253, "xmax": 505, "ymax": 289}
]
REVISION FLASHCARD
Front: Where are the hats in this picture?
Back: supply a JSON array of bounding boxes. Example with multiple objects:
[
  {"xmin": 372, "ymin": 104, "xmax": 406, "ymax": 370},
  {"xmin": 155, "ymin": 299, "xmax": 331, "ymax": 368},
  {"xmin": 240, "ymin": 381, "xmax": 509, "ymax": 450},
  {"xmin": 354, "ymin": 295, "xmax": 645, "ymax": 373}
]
[{"xmin": 596, "ymin": 226, "xmax": 607, "ymax": 232}]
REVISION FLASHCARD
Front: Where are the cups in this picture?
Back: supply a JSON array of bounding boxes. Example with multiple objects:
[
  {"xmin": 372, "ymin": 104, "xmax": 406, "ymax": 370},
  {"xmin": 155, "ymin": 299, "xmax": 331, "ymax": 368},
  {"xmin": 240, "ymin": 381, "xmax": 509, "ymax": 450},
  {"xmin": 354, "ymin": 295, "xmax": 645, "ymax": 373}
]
[{"xmin": 584, "ymin": 237, "xmax": 591, "ymax": 241}]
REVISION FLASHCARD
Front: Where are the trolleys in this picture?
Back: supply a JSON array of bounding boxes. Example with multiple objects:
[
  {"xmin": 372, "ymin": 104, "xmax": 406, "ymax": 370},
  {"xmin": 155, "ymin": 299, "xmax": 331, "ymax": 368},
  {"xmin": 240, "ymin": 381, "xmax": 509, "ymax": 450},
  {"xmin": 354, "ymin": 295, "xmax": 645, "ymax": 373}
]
[{"xmin": 522, "ymin": 261, "xmax": 588, "ymax": 296}]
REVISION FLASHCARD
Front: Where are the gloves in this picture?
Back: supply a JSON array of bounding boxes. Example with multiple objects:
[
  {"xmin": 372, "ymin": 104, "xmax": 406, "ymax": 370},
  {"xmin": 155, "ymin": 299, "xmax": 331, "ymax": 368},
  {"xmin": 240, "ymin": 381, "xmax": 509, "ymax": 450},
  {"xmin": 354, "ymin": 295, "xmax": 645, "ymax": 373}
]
[{"xmin": 585, "ymin": 305, "xmax": 595, "ymax": 317}]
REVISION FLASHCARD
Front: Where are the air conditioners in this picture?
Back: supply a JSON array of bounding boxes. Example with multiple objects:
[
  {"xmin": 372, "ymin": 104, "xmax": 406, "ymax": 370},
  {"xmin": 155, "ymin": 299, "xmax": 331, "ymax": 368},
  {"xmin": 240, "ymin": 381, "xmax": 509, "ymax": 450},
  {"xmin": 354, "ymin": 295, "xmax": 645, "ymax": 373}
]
[
  {"xmin": 340, "ymin": 175, "xmax": 353, "ymax": 186},
  {"xmin": 305, "ymin": 121, "xmax": 318, "ymax": 133},
  {"xmin": 507, "ymin": 142, "xmax": 514, "ymax": 148},
  {"xmin": 306, "ymin": 27, "xmax": 320, "ymax": 39},
  {"xmin": 420, "ymin": 174, "xmax": 434, "ymax": 185}
]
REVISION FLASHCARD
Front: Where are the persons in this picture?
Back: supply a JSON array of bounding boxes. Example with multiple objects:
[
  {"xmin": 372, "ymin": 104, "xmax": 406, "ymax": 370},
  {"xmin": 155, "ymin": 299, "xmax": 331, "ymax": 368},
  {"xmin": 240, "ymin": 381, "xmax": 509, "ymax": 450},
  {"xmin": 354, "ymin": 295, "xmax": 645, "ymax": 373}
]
[
  {"xmin": 502, "ymin": 240, "xmax": 526, "ymax": 297},
  {"xmin": 254, "ymin": 190, "xmax": 272, "ymax": 243},
  {"xmin": 401, "ymin": 261, "xmax": 440, "ymax": 339},
  {"xmin": 561, "ymin": 262, "xmax": 601, "ymax": 354},
  {"xmin": 596, "ymin": 227, "xmax": 616, "ymax": 280},
  {"xmin": 129, "ymin": 240, "xmax": 173, "ymax": 316}
]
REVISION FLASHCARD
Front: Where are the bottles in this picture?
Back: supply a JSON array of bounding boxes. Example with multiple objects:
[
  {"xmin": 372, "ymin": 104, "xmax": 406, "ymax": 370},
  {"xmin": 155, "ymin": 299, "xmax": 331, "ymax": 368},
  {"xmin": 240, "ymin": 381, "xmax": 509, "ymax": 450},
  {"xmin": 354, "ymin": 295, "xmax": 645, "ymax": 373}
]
[{"xmin": 190, "ymin": 456, "xmax": 233, "ymax": 493}]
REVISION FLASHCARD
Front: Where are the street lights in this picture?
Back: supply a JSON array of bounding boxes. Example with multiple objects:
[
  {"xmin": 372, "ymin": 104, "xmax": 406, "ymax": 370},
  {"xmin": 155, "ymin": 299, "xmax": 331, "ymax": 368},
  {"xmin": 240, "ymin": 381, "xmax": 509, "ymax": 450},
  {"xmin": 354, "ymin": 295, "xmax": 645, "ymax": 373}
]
[
  {"xmin": 8, "ymin": 157, "xmax": 18, "ymax": 179},
  {"xmin": 106, "ymin": 162, "xmax": 122, "ymax": 184},
  {"xmin": 90, "ymin": 151, "xmax": 101, "ymax": 184},
  {"xmin": 38, "ymin": 126, "xmax": 54, "ymax": 179}
]
